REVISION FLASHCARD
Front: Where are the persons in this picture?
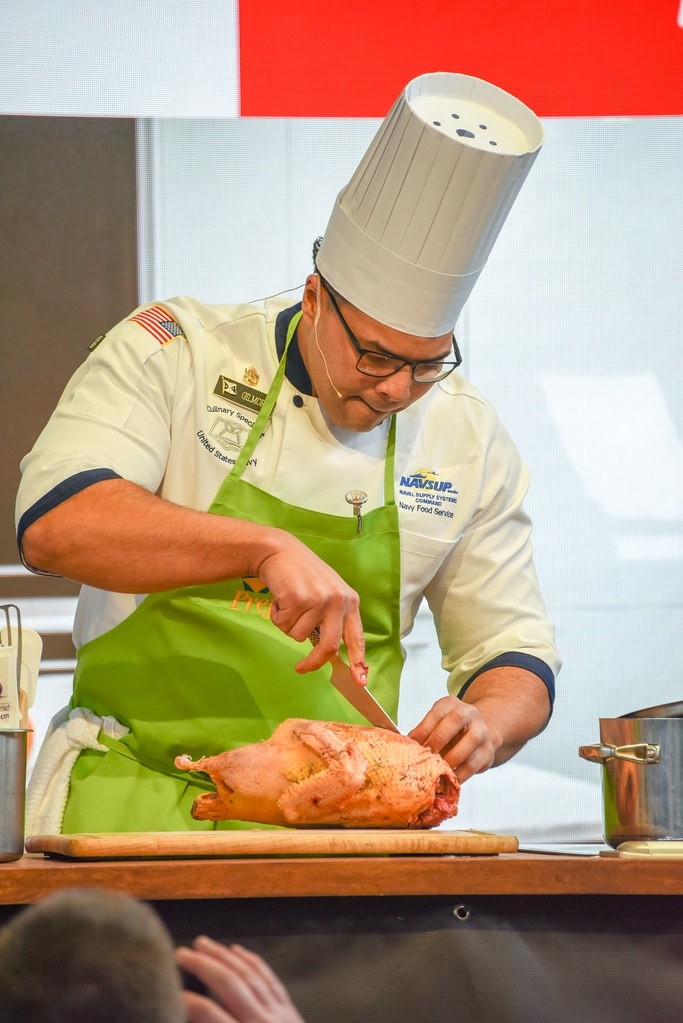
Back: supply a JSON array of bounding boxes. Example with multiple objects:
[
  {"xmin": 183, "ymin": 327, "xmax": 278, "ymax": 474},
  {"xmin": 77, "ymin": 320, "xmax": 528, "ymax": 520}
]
[
  {"xmin": 15, "ymin": 70, "xmax": 567, "ymax": 841},
  {"xmin": 1, "ymin": 886, "xmax": 306, "ymax": 1023}
]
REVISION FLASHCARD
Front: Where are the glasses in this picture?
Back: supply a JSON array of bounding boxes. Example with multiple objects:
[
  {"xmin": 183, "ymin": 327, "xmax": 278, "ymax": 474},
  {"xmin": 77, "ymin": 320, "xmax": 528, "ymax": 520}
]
[{"xmin": 321, "ymin": 282, "xmax": 462, "ymax": 384}]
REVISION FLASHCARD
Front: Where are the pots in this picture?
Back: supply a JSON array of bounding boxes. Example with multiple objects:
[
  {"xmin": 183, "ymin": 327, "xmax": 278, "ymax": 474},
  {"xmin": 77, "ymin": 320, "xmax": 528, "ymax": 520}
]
[
  {"xmin": 0, "ymin": 729, "xmax": 35, "ymax": 864},
  {"xmin": 578, "ymin": 699, "xmax": 683, "ymax": 850}
]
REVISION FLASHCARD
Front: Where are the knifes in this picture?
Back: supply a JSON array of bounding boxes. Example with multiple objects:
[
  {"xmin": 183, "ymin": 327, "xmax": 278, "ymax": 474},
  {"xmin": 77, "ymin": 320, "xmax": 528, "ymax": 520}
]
[{"xmin": 308, "ymin": 628, "xmax": 403, "ymax": 733}]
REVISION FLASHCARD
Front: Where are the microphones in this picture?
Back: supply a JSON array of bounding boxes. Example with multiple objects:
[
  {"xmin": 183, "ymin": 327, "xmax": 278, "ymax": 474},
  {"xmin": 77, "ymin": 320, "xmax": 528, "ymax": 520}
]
[{"xmin": 314, "ymin": 272, "xmax": 343, "ymax": 400}]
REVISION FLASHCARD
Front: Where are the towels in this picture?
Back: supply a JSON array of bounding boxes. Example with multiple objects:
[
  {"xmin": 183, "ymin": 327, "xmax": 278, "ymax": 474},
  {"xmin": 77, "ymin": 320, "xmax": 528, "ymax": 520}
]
[{"xmin": 11, "ymin": 707, "xmax": 133, "ymax": 851}]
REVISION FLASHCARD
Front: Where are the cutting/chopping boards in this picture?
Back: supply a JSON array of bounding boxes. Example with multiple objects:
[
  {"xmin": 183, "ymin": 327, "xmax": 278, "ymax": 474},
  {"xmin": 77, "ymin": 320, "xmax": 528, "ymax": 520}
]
[{"xmin": 25, "ymin": 830, "xmax": 518, "ymax": 859}]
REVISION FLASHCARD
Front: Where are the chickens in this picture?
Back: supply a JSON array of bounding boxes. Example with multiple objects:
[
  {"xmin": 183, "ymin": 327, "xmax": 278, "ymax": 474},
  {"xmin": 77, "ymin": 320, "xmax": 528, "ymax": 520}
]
[{"xmin": 174, "ymin": 718, "xmax": 462, "ymax": 830}]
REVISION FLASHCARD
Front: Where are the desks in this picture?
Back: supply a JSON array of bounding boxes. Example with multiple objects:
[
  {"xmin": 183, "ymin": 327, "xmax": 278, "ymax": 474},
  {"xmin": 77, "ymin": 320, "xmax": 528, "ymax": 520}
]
[{"xmin": 0, "ymin": 835, "xmax": 683, "ymax": 1023}]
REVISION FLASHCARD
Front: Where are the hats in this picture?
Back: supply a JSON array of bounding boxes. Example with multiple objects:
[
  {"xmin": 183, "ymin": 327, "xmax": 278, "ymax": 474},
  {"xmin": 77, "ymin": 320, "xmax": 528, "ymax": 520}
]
[{"xmin": 315, "ymin": 72, "xmax": 545, "ymax": 338}]
